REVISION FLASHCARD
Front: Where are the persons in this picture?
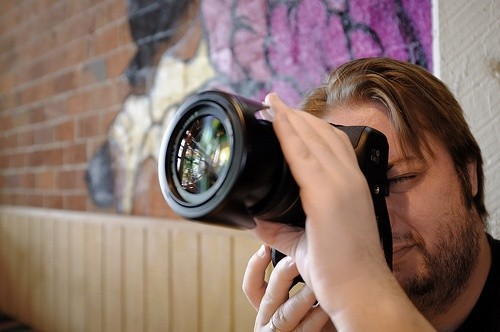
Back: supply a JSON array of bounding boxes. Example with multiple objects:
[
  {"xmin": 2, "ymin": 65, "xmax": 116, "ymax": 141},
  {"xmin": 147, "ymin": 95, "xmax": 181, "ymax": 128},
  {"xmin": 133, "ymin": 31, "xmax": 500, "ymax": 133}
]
[{"xmin": 242, "ymin": 57, "xmax": 500, "ymax": 332}]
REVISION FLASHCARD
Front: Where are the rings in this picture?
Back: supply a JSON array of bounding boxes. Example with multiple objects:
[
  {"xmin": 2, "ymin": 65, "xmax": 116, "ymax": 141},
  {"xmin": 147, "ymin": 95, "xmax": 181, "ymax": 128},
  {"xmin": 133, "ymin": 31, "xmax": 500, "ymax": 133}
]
[{"xmin": 270, "ymin": 318, "xmax": 282, "ymax": 332}]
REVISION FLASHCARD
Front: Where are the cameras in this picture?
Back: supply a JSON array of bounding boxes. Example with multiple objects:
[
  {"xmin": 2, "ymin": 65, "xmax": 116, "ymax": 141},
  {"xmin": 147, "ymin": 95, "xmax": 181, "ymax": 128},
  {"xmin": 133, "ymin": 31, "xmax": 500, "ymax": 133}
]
[{"xmin": 157, "ymin": 90, "xmax": 389, "ymax": 284}]
